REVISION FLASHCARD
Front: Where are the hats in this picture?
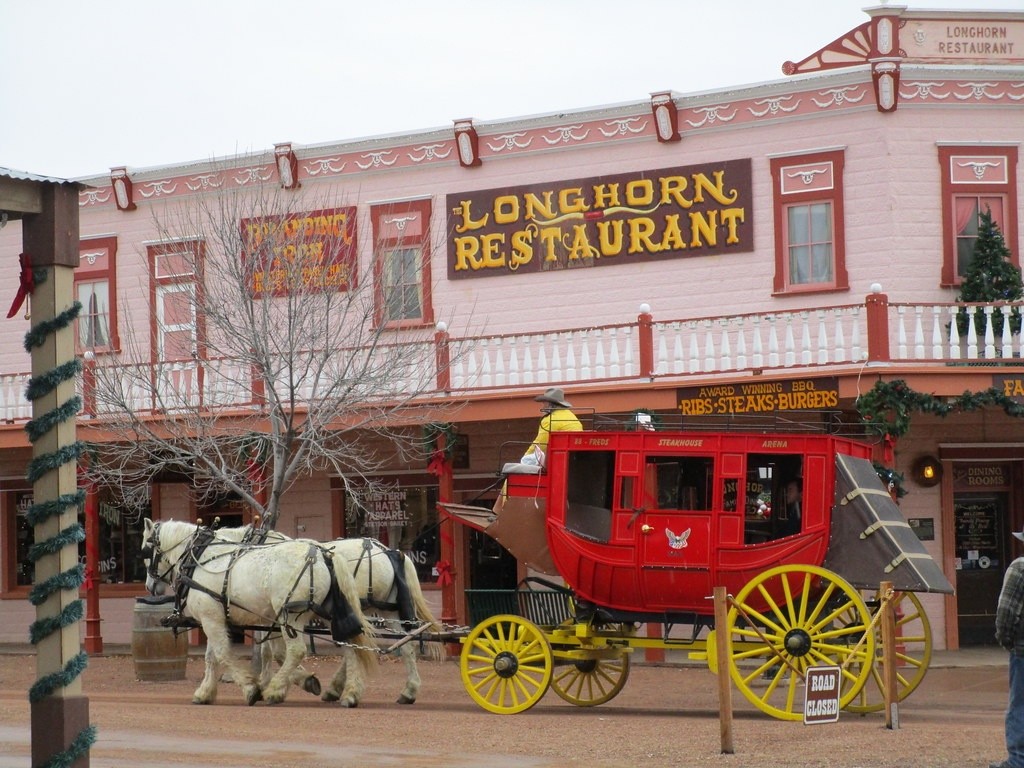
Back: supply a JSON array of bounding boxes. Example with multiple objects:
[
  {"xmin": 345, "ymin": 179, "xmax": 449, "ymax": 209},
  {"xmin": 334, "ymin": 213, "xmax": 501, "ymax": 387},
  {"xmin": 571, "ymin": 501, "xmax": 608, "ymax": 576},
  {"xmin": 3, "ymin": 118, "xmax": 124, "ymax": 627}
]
[
  {"xmin": 534, "ymin": 388, "xmax": 572, "ymax": 408},
  {"xmin": 1012, "ymin": 532, "xmax": 1024, "ymax": 541}
]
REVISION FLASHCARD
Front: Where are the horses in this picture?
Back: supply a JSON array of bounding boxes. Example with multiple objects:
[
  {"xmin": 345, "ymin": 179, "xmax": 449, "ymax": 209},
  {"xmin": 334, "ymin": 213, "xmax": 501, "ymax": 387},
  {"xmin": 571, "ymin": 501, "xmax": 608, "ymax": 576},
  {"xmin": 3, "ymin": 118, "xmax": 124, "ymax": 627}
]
[{"xmin": 140, "ymin": 518, "xmax": 447, "ymax": 708}]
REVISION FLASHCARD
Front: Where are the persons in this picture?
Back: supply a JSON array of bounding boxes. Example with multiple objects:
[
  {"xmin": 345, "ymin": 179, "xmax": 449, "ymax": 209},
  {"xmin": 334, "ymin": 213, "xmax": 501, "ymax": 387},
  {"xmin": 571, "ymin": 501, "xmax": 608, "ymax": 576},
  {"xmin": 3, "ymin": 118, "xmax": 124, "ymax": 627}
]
[
  {"xmin": 988, "ymin": 525, "xmax": 1024, "ymax": 768},
  {"xmin": 766, "ymin": 474, "xmax": 803, "ymax": 544},
  {"xmin": 414, "ymin": 525, "xmax": 436, "ymax": 581},
  {"xmin": 488, "ymin": 387, "xmax": 584, "ymax": 523}
]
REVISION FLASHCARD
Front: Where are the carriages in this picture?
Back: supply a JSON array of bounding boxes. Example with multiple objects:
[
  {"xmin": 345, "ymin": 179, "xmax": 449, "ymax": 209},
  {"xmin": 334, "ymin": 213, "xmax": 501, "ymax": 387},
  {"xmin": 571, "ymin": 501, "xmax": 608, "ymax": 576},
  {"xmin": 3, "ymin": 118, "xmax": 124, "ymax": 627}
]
[{"xmin": 140, "ymin": 408, "xmax": 953, "ymax": 722}]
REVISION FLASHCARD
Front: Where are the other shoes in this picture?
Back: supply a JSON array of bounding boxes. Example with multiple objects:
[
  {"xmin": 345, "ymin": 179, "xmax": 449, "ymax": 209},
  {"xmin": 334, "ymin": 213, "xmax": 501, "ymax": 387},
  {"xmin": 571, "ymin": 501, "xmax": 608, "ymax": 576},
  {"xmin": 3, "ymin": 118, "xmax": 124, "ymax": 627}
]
[
  {"xmin": 488, "ymin": 517, "xmax": 497, "ymax": 523},
  {"xmin": 989, "ymin": 762, "xmax": 1005, "ymax": 768}
]
